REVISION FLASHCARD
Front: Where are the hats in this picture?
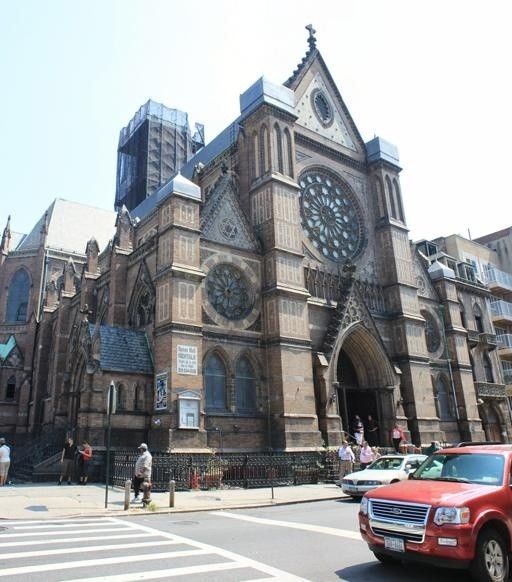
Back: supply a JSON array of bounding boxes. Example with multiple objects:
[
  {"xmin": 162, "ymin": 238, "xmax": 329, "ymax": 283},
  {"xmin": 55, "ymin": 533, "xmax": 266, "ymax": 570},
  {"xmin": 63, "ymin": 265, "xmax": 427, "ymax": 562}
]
[{"xmin": 137, "ymin": 443, "xmax": 148, "ymax": 450}]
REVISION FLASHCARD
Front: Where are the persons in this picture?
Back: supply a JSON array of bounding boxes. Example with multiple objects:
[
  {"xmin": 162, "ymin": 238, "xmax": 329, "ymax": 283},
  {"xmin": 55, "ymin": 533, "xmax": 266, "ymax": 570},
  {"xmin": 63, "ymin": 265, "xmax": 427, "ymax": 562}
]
[
  {"xmin": 392, "ymin": 423, "xmax": 407, "ymax": 455},
  {"xmin": 353, "ymin": 415, "xmax": 365, "ymax": 446},
  {"xmin": 335, "ymin": 440, "xmax": 356, "ymax": 488},
  {"xmin": 57, "ymin": 435, "xmax": 77, "ymax": 485},
  {"xmin": 359, "ymin": 438, "xmax": 372, "ymax": 470},
  {"xmin": 365, "ymin": 414, "xmax": 379, "ymax": 446},
  {"xmin": 370, "ymin": 446, "xmax": 380, "ymax": 460},
  {"xmin": 0, "ymin": 438, "xmax": 11, "ymax": 486},
  {"xmin": 130, "ymin": 442, "xmax": 153, "ymax": 504},
  {"xmin": 77, "ymin": 440, "xmax": 92, "ymax": 485},
  {"xmin": 0, "ymin": 435, "xmax": 17, "ymax": 484}
]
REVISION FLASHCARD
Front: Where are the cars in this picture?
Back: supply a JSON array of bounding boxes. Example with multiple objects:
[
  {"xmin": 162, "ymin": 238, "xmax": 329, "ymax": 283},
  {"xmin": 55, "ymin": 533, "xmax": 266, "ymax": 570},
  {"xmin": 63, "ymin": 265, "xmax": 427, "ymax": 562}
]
[{"xmin": 341, "ymin": 454, "xmax": 456, "ymax": 502}]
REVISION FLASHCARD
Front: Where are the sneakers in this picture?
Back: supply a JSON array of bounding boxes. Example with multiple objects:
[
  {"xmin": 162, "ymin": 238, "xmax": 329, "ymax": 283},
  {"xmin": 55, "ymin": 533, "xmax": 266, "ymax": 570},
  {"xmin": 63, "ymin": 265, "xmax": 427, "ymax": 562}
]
[
  {"xmin": 132, "ymin": 497, "xmax": 139, "ymax": 501},
  {"xmin": 58, "ymin": 481, "xmax": 87, "ymax": 484}
]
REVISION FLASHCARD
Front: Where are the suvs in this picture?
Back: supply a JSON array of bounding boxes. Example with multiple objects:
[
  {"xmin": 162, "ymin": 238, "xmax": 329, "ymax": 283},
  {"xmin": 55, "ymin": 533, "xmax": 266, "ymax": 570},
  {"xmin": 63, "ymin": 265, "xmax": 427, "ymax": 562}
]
[{"xmin": 358, "ymin": 441, "xmax": 512, "ymax": 582}]
